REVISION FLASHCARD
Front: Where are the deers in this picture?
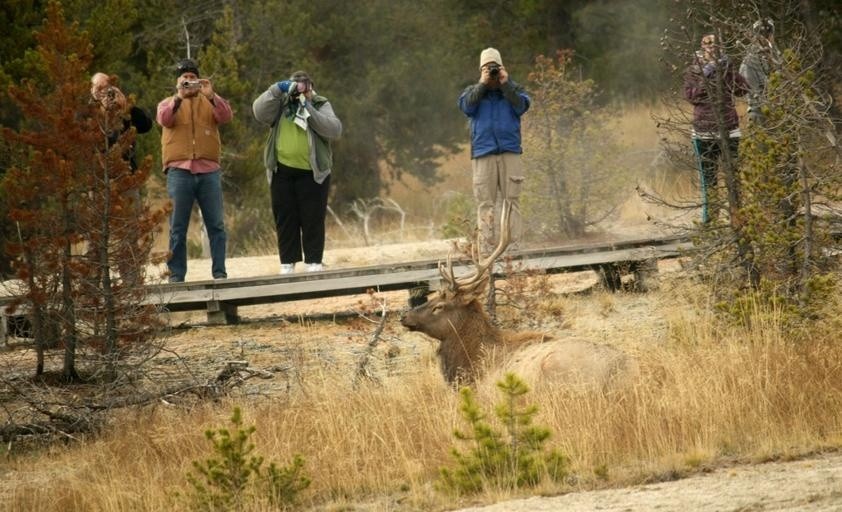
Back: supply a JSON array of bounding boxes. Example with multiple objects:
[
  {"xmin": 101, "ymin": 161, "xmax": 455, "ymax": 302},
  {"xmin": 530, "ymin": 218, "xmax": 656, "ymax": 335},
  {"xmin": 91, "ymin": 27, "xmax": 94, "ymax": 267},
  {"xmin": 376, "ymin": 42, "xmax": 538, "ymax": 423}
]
[{"xmin": 399, "ymin": 199, "xmax": 641, "ymax": 397}]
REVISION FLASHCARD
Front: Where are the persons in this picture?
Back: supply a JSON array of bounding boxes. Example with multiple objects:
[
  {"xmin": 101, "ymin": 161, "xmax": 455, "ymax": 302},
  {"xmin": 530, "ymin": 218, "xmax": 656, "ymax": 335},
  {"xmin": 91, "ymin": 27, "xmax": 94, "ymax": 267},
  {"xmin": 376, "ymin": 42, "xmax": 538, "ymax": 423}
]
[
  {"xmin": 156, "ymin": 60, "xmax": 234, "ymax": 283},
  {"xmin": 81, "ymin": 72, "xmax": 152, "ymax": 305},
  {"xmin": 252, "ymin": 72, "xmax": 342, "ymax": 274},
  {"xmin": 685, "ymin": 33, "xmax": 749, "ymax": 225},
  {"xmin": 738, "ymin": 17, "xmax": 784, "ymax": 128},
  {"xmin": 458, "ymin": 48, "xmax": 530, "ymax": 257}
]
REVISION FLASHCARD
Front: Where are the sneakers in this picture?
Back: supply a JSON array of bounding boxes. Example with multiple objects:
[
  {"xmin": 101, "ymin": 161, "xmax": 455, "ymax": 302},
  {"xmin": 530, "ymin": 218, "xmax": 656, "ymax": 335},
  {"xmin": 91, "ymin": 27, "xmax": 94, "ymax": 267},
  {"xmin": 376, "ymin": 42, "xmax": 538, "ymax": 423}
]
[
  {"xmin": 308, "ymin": 263, "xmax": 323, "ymax": 272},
  {"xmin": 279, "ymin": 263, "xmax": 295, "ymax": 274}
]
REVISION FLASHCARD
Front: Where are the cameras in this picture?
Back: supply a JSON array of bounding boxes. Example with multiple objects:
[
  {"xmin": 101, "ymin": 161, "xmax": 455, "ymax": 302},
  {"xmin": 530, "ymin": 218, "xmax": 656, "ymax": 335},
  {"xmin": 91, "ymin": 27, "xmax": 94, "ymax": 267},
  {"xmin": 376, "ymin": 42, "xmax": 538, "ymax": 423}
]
[
  {"xmin": 488, "ymin": 64, "xmax": 501, "ymax": 80},
  {"xmin": 289, "ymin": 92, "xmax": 301, "ymax": 104},
  {"xmin": 182, "ymin": 81, "xmax": 201, "ymax": 88}
]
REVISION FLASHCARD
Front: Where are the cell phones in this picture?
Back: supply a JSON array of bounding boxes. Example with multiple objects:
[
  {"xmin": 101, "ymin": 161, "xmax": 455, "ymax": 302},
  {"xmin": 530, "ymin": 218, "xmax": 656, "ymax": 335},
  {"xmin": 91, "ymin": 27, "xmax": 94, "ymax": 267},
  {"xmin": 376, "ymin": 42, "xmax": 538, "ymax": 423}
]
[{"xmin": 107, "ymin": 88, "xmax": 116, "ymax": 104}]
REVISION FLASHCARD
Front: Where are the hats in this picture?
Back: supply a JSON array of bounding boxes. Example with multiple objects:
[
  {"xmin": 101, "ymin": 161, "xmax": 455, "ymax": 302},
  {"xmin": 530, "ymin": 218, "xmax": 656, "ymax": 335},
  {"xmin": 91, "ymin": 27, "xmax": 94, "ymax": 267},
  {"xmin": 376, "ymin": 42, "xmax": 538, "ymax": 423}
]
[
  {"xmin": 289, "ymin": 71, "xmax": 315, "ymax": 90},
  {"xmin": 176, "ymin": 58, "xmax": 200, "ymax": 80},
  {"xmin": 479, "ymin": 47, "xmax": 504, "ymax": 71},
  {"xmin": 751, "ymin": 18, "xmax": 775, "ymax": 39}
]
